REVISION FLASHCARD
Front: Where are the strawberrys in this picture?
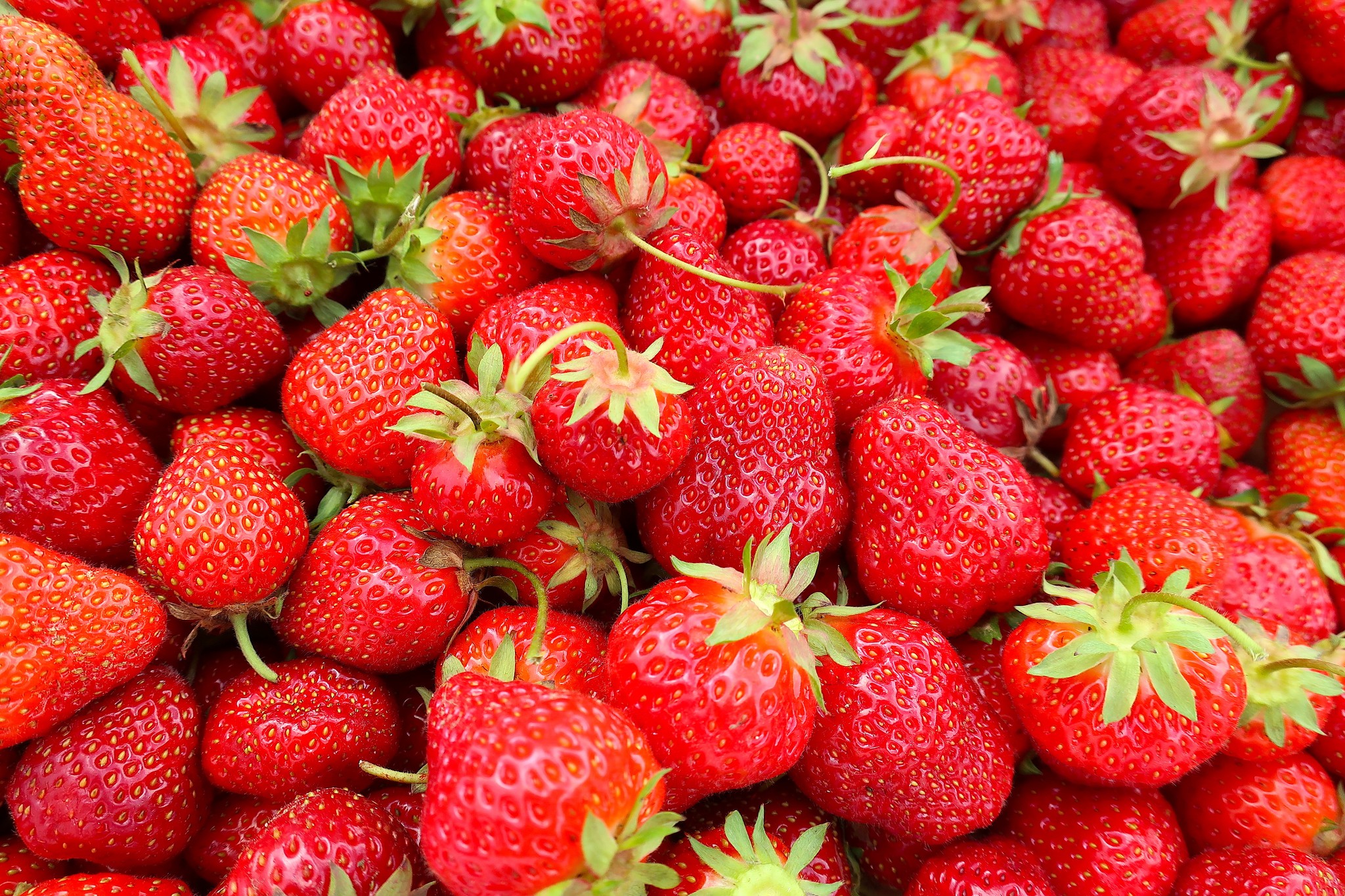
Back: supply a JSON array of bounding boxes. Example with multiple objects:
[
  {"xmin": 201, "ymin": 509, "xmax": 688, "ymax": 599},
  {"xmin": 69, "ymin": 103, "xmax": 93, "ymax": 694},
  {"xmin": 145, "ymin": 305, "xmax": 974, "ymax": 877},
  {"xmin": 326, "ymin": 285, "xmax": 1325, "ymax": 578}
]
[{"xmin": 0, "ymin": 0, "xmax": 1345, "ymax": 896}]
